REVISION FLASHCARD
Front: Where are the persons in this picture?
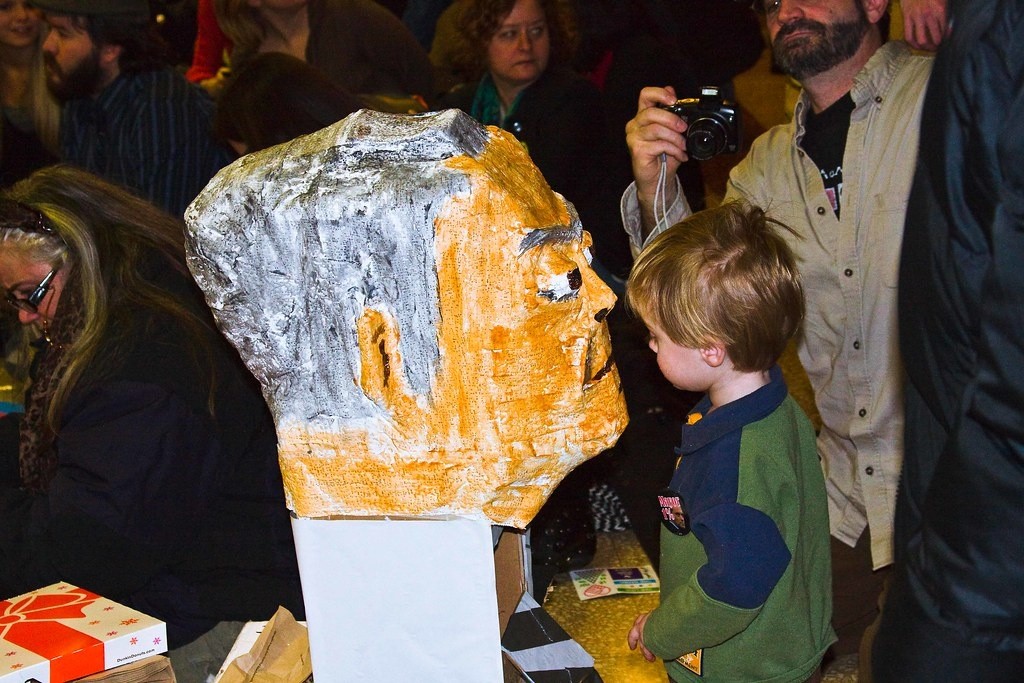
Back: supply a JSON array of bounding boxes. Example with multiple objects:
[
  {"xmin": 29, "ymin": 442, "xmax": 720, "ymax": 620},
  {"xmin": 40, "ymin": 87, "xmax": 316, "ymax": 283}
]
[
  {"xmin": 872, "ymin": 2, "xmax": 1024, "ymax": 683},
  {"xmin": 182, "ymin": 107, "xmax": 629, "ymax": 532},
  {"xmin": 614, "ymin": 569, "xmax": 642, "ymax": 578},
  {"xmin": 626, "ymin": 202, "xmax": 842, "ymax": 681},
  {"xmin": 668, "ymin": 507, "xmax": 685, "ymax": 530},
  {"xmin": 0, "ymin": 169, "xmax": 305, "ymax": 653},
  {"xmin": 618, "ymin": 1, "xmax": 949, "ymax": 658},
  {"xmin": 1, "ymin": 1, "xmax": 638, "ymax": 279}
]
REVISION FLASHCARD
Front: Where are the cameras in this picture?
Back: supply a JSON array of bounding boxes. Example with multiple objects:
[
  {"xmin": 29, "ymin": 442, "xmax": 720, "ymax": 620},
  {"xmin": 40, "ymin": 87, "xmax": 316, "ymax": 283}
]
[{"xmin": 660, "ymin": 86, "xmax": 740, "ymax": 161}]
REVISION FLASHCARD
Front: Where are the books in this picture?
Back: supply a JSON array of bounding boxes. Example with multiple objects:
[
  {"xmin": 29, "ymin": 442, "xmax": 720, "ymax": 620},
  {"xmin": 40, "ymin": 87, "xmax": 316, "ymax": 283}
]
[{"xmin": 0, "ymin": 581, "xmax": 177, "ymax": 683}]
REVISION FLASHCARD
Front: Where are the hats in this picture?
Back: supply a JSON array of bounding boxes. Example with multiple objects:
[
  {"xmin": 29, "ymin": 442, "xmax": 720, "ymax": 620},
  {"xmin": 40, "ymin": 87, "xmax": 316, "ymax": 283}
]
[{"xmin": 30, "ymin": 0, "xmax": 152, "ymax": 21}]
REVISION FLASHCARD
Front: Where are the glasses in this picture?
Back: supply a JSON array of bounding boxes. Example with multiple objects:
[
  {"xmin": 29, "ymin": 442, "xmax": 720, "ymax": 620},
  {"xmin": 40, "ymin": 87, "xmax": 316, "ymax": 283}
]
[
  {"xmin": 748, "ymin": 0, "xmax": 781, "ymax": 15},
  {"xmin": 7, "ymin": 268, "xmax": 58, "ymax": 313}
]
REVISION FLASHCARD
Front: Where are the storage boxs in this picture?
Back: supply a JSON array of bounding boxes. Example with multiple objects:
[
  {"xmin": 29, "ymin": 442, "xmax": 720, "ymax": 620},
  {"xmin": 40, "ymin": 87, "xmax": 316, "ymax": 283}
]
[{"xmin": 0, "ymin": 582, "xmax": 167, "ymax": 682}]
